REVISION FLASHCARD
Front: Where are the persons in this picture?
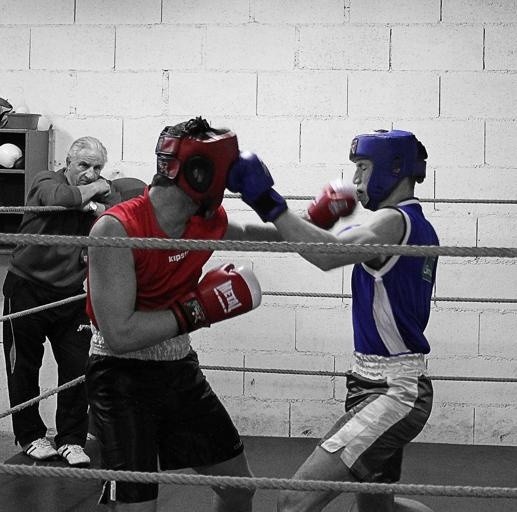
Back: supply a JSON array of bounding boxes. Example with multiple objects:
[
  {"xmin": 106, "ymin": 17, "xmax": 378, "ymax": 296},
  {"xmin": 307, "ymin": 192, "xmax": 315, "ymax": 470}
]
[
  {"xmin": 225, "ymin": 128, "xmax": 440, "ymax": 510},
  {"xmin": 1, "ymin": 136, "xmax": 122, "ymax": 467},
  {"xmin": 86, "ymin": 115, "xmax": 360, "ymax": 512}
]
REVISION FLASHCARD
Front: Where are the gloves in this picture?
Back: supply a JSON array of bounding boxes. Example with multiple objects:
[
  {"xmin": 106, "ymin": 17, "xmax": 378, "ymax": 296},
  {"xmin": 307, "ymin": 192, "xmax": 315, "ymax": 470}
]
[
  {"xmin": 168, "ymin": 262, "xmax": 261, "ymax": 335},
  {"xmin": 307, "ymin": 184, "xmax": 356, "ymax": 230},
  {"xmin": 0, "ymin": 144, "xmax": 24, "ymax": 169},
  {"xmin": 226, "ymin": 152, "xmax": 287, "ymax": 223}
]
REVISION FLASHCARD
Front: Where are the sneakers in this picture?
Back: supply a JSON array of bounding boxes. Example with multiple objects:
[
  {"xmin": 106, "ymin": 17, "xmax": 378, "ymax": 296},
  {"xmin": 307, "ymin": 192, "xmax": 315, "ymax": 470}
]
[
  {"xmin": 56, "ymin": 444, "xmax": 91, "ymax": 465},
  {"xmin": 15, "ymin": 436, "xmax": 58, "ymax": 459}
]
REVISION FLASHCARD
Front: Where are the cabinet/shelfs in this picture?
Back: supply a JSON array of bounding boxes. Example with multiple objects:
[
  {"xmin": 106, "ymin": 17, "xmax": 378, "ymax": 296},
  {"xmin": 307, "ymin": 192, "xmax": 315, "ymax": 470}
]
[{"xmin": 0, "ymin": 129, "xmax": 53, "ymax": 255}]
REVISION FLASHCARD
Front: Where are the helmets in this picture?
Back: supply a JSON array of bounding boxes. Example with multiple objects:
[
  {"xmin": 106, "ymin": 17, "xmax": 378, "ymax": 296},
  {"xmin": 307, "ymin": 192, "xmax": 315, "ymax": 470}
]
[
  {"xmin": 155, "ymin": 117, "xmax": 238, "ymax": 220},
  {"xmin": 348, "ymin": 130, "xmax": 427, "ymax": 211}
]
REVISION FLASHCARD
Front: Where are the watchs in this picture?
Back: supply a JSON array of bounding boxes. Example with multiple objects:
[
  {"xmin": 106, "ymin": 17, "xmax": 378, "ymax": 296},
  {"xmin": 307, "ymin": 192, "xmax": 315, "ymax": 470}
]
[{"xmin": 86, "ymin": 200, "xmax": 98, "ymax": 215}]
